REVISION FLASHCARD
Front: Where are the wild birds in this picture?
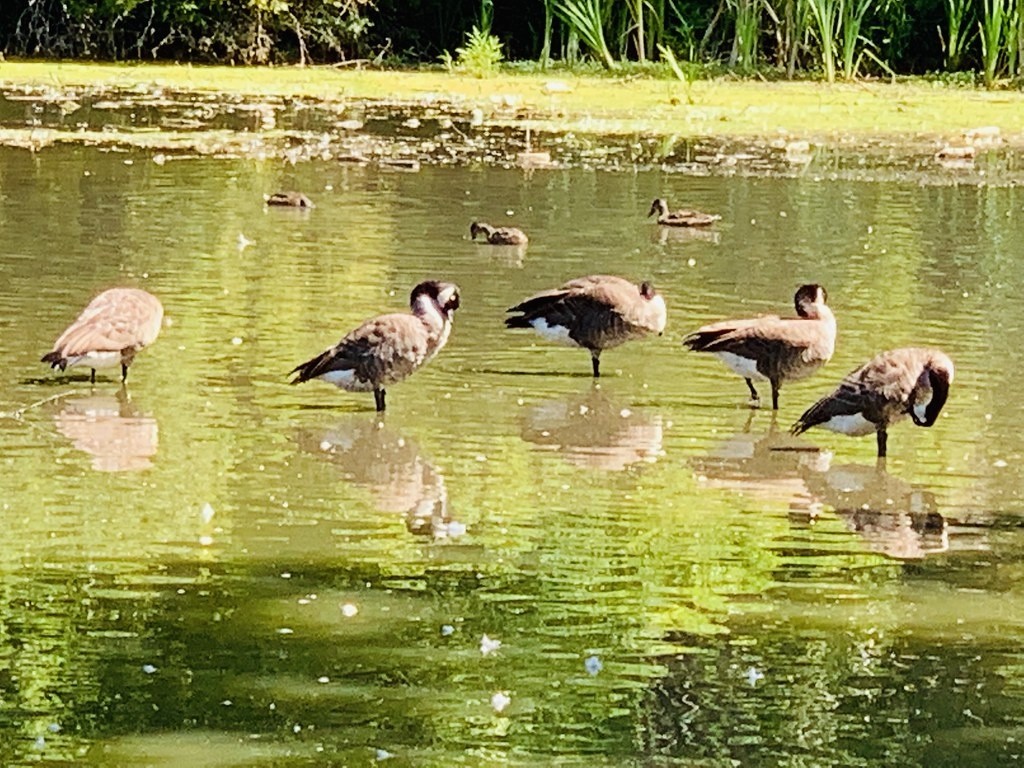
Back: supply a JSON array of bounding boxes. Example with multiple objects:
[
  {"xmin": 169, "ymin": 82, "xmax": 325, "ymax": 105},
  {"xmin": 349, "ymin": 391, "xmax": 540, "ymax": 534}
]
[
  {"xmin": 648, "ymin": 199, "xmax": 722, "ymax": 226},
  {"xmin": 504, "ymin": 274, "xmax": 667, "ymax": 377},
  {"xmin": 53, "ymin": 380, "xmax": 158, "ymax": 473},
  {"xmin": 284, "ymin": 411, "xmax": 467, "ymax": 538},
  {"xmin": 266, "ymin": 191, "xmax": 311, "ymax": 207},
  {"xmin": 285, "ymin": 281, "xmax": 461, "ymax": 412},
  {"xmin": 471, "ymin": 222, "xmax": 528, "ymax": 244},
  {"xmin": 41, "ymin": 287, "xmax": 164, "ymax": 383},
  {"xmin": 788, "ymin": 346, "xmax": 954, "ymax": 456},
  {"xmin": 651, "ymin": 224, "xmax": 722, "ymax": 247},
  {"xmin": 800, "ymin": 456, "xmax": 949, "ymax": 559},
  {"xmin": 680, "ymin": 284, "xmax": 837, "ymax": 410},
  {"xmin": 518, "ymin": 380, "xmax": 668, "ymax": 474},
  {"xmin": 687, "ymin": 407, "xmax": 833, "ymax": 525}
]
